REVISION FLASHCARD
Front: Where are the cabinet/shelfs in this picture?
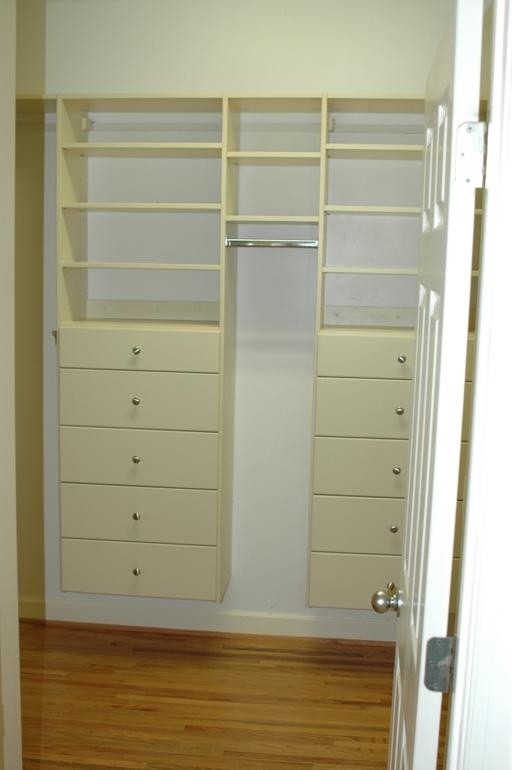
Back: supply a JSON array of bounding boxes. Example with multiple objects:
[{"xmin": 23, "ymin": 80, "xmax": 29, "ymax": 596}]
[{"xmin": 44, "ymin": 93, "xmax": 488, "ymax": 643}]
